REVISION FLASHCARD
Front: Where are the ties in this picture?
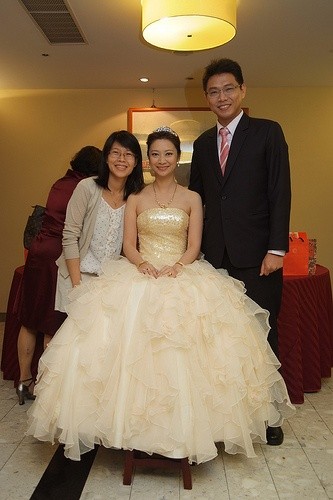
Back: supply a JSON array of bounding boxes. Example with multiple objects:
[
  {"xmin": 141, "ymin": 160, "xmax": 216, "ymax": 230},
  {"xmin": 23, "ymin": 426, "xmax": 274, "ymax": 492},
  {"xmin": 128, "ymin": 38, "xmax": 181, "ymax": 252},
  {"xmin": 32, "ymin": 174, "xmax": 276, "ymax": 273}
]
[{"xmin": 219, "ymin": 127, "xmax": 230, "ymax": 176}]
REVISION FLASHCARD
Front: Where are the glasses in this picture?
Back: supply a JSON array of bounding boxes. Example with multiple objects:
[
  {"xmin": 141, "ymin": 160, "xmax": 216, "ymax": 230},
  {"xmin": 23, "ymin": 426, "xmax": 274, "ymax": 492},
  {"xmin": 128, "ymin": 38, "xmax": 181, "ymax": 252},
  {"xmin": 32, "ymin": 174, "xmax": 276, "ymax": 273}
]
[
  {"xmin": 206, "ymin": 82, "xmax": 242, "ymax": 96},
  {"xmin": 110, "ymin": 151, "xmax": 137, "ymax": 157}
]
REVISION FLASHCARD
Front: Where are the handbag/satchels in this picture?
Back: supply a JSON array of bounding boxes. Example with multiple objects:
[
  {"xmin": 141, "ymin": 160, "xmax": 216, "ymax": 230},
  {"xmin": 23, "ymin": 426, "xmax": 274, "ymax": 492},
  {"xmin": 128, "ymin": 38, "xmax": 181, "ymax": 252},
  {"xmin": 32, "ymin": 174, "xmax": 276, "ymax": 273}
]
[
  {"xmin": 282, "ymin": 232, "xmax": 318, "ymax": 276},
  {"xmin": 23, "ymin": 204, "xmax": 46, "ymax": 250}
]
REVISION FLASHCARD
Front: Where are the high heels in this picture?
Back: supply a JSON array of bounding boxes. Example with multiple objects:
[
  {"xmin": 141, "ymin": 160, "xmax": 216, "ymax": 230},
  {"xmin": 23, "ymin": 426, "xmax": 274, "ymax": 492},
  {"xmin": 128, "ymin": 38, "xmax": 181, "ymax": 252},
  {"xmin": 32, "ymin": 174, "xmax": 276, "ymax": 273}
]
[{"xmin": 16, "ymin": 378, "xmax": 36, "ymax": 405}]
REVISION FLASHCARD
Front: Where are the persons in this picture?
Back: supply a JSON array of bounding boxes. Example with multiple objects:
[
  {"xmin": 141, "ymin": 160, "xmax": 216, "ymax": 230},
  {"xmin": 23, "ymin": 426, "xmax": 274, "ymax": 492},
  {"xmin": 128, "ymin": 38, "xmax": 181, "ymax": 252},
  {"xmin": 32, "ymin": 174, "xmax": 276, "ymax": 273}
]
[
  {"xmin": 188, "ymin": 59, "xmax": 292, "ymax": 446},
  {"xmin": 122, "ymin": 126, "xmax": 202, "ymax": 465},
  {"xmin": 17, "ymin": 146, "xmax": 102, "ymax": 405},
  {"xmin": 55, "ymin": 130, "xmax": 144, "ymax": 313}
]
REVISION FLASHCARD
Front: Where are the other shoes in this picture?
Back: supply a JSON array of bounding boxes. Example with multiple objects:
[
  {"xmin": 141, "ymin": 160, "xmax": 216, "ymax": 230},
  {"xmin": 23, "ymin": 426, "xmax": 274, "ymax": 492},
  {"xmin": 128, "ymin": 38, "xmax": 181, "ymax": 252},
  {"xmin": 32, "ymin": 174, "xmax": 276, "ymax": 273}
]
[{"xmin": 266, "ymin": 426, "xmax": 284, "ymax": 445}]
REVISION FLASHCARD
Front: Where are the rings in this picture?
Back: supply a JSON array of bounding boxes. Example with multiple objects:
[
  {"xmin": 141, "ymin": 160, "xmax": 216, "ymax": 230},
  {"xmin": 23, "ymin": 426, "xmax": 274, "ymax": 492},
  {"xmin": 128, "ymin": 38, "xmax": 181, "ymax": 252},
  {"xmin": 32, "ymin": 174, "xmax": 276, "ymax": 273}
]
[{"xmin": 169, "ymin": 272, "xmax": 172, "ymax": 275}]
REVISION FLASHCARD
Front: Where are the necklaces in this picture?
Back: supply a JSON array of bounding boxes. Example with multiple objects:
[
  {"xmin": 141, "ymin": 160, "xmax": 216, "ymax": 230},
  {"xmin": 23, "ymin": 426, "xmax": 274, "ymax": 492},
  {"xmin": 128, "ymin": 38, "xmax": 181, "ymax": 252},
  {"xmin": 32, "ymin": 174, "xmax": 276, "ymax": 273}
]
[
  {"xmin": 153, "ymin": 180, "xmax": 177, "ymax": 208},
  {"xmin": 111, "ymin": 187, "xmax": 124, "ymax": 207}
]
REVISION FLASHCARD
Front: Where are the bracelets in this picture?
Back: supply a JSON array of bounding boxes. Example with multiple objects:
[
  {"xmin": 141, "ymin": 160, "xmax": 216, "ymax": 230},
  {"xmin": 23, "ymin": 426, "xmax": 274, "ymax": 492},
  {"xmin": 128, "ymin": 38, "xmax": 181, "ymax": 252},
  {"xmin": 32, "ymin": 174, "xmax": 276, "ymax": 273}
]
[
  {"xmin": 138, "ymin": 260, "xmax": 148, "ymax": 268},
  {"xmin": 176, "ymin": 262, "xmax": 183, "ymax": 267}
]
